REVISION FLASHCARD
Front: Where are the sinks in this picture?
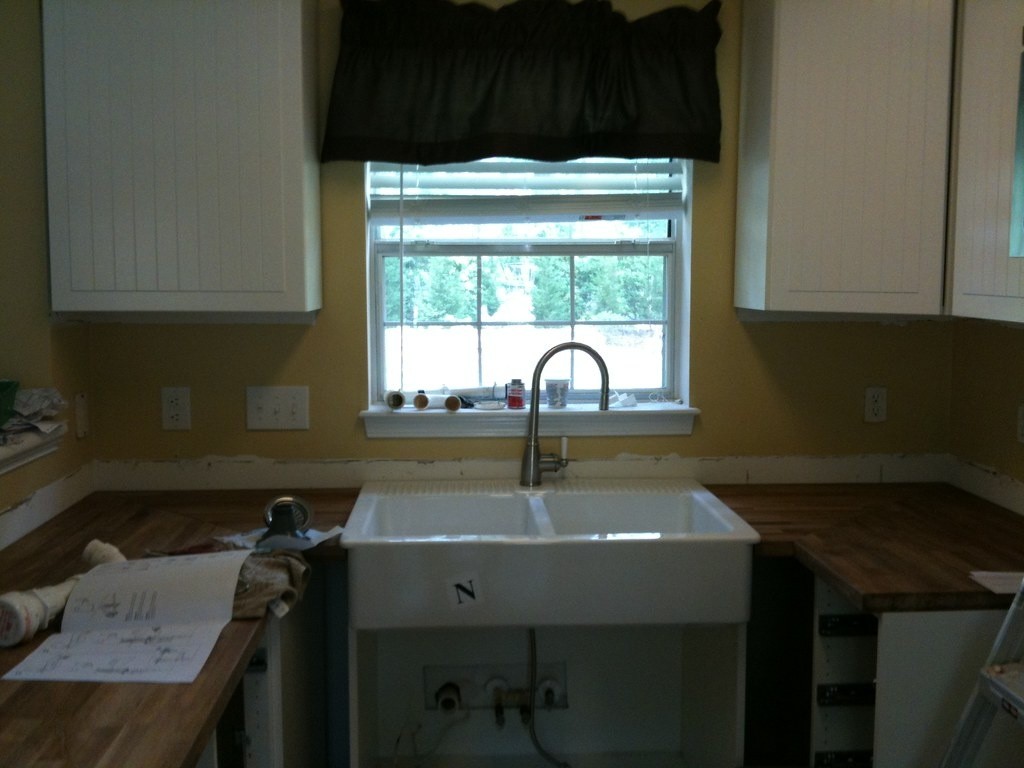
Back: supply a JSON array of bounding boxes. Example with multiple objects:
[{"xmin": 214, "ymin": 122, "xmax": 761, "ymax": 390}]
[
  {"xmin": 338, "ymin": 494, "xmax": 542, "ymax": 627},
  {"xmin": 542, "ymin": 492, "xmax": 761, "ymax": 627}
]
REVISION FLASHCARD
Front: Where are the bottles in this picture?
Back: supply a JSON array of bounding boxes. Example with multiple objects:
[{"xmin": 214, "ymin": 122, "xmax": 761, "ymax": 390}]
[{"xmin": 507, "ymin": 379, "xmax": 525, "ymax": 408}]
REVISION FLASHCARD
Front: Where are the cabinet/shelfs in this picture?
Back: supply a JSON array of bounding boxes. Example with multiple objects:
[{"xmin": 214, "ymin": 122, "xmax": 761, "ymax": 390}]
[
  {"xmin": 39, "ymin": 0, "xmax": 323, "ymax": 328},
  {"xmin": 942, "ymin": 0, "xmax": 1024, "ymax": 330},
  {"xmin": 732, "ymin": 0, "xmax": 955, "ymax": 324}
]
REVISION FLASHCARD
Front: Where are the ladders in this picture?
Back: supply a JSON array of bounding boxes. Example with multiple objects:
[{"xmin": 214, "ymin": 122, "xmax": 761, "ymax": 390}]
[{"xmin": 939, "ymin": 578, "xmax": 1024, "ymax": 767}]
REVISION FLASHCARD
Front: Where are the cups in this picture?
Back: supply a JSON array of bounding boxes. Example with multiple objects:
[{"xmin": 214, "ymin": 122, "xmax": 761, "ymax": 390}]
[{"xmin": 545, "ymin": 378, "xmax": 569, "ymax": 409}]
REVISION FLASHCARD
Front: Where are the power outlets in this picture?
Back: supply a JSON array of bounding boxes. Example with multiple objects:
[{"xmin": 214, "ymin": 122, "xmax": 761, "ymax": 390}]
[
  {"xmin": 72, "ymin": 392, "xmax": 90, "ymax": 439},
  {"xmin": 1016, "ymin": 405, "xmax": 1024, "ymax": 443},
  {"xmin": 864, "ymin": 387, "xmax": 888, "ymax": 422},
  {"xmin": 162, "ymin": 386, "xmax": 192, "ymax": 433}
]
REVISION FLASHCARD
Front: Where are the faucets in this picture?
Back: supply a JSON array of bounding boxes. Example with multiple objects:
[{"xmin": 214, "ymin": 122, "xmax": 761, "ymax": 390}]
[{"xmin": 520, "ymin": 341, "xmax": 610, "ymax": 486}]
